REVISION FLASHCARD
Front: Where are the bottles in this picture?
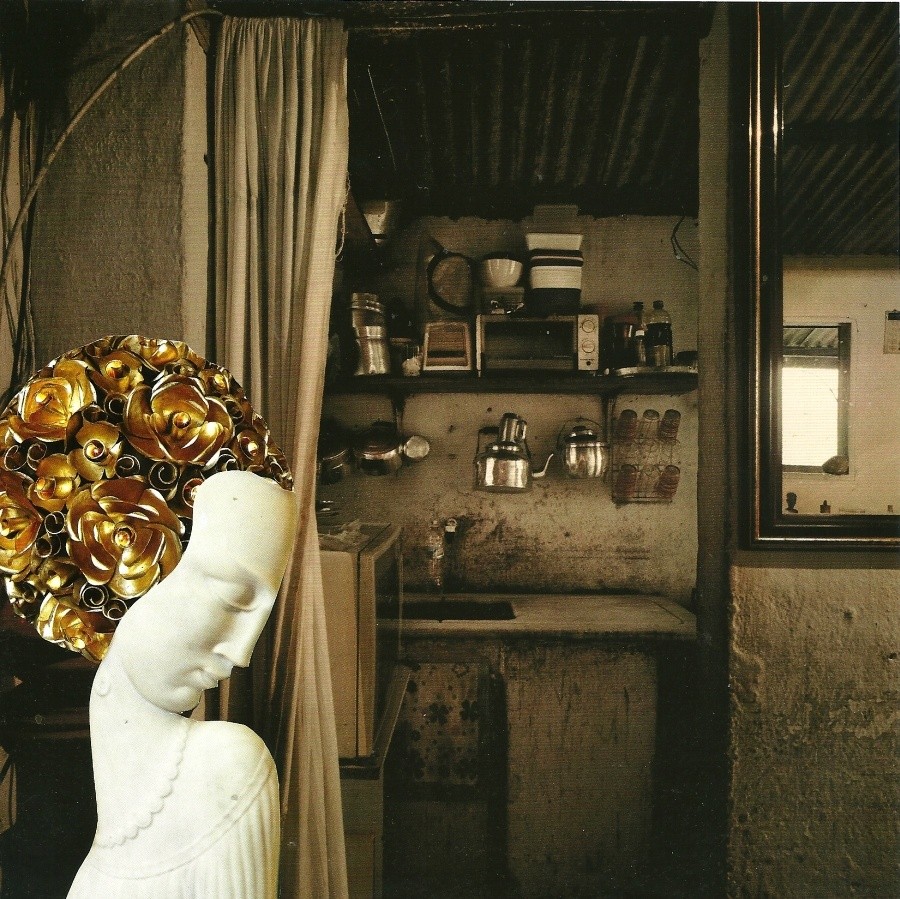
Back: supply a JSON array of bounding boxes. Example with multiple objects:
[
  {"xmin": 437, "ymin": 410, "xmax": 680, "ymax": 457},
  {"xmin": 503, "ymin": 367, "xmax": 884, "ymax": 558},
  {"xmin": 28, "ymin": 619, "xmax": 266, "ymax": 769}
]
[
  {"xmin": 622, "ymin": 299, "xmax": 674, "ymax": 366},
  {"xmin": 425, "ymin": 521, "xmax": 444, "ymax": 592}
]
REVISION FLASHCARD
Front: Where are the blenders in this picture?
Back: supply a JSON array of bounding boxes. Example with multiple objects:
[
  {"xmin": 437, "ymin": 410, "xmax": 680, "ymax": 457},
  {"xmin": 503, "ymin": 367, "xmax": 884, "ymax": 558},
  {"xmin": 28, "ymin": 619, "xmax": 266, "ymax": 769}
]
[{"xmin": 421, "ymin": 255, "xmax": 472, "ymax": 373}]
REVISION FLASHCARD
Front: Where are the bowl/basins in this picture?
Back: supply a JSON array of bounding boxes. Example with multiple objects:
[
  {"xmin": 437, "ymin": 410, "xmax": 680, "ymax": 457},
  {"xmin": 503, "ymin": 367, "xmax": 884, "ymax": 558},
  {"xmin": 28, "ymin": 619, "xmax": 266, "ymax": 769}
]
[{"xmin": 478, "ymin": 257, "xmax": 522, "ymax": 288}]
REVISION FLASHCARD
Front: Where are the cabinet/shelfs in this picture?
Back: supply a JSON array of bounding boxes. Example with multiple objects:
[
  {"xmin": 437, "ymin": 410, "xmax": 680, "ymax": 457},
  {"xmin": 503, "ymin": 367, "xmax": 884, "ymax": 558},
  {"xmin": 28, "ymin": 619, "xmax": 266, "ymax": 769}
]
[{"xmin": 605, "ymin": 395, "xmax": 682, "ymax": 506}]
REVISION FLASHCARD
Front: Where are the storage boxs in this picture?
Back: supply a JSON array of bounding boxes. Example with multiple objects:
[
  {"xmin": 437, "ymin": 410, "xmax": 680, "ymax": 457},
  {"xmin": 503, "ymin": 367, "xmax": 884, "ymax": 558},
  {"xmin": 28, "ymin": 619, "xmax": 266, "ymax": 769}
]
[{"xmin": 525, "ymin": 230, "xmax": 583, "ymax": 314}]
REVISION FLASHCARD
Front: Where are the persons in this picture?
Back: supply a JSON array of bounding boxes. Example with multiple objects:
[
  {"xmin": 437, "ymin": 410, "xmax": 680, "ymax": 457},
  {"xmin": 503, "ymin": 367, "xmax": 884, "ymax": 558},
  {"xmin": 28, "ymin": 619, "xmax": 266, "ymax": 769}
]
[{"xmin": 0, "ymin": 334, "xmax": 300, "ymax": 899}]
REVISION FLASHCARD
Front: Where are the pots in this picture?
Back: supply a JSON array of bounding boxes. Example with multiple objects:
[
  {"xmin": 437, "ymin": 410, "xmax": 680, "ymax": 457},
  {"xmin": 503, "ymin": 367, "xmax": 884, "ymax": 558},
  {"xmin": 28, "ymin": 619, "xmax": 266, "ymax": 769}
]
[
  {"xmin": 317, "ymin": 424, "xmax": 406, "ymax": 480},
  {"xmin": 339, "ymin": 293, "xmax": 390, "ymax": 376},
  {"xmin": 558, "ymin": 416, "xmax": 609, "ymax": 479}
]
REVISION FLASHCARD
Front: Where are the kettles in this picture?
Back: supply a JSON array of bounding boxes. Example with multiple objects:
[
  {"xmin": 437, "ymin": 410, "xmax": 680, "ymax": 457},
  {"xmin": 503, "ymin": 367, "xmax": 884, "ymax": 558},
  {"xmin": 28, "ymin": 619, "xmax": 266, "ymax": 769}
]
[{"xmin": 472, "ymin": 424, "xmax": 557, "ymax": 494}]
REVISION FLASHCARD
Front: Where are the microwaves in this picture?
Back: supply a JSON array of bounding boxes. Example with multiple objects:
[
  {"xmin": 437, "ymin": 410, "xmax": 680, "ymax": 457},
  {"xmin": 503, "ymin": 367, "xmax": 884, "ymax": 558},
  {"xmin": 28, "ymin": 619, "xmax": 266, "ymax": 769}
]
[{"xmin": 475, "ymin": 313, "xmax": 597, "ymax": 379}]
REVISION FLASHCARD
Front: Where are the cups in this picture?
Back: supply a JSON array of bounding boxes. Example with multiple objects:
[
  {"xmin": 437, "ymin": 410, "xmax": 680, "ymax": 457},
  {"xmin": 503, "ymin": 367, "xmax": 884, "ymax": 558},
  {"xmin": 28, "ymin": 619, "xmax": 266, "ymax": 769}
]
[
  {"xmin": 401, "ymin": 344, "xmax": 421, "ymax": 380},
  {"xmin": 641, "ymin": 409, "xmax": 659, "ymax": 446},
  {"xmin": 658, "ymin": 464, "xmax": 680, "ymax": 497},
  {"xmin": 637, "ymin": 465, "xmax": 658, "ymax": 496},
  {"xmin": 618, "ymin": 463, "xmax": 636, "ymax": 497},
  {"xmin": 661, "ymin": 406, "xmax": 681, "ymax": 447},
  {"xmin": 617, "ymin": 409, "xmax": 637, "ymax": 443},
  {"xmin": 405, "ymin": 435, "xmax": 429, "ymax": 462}
]
